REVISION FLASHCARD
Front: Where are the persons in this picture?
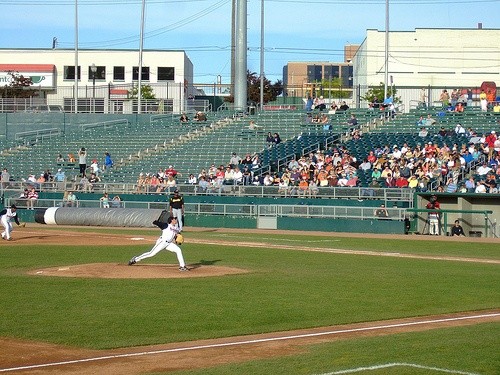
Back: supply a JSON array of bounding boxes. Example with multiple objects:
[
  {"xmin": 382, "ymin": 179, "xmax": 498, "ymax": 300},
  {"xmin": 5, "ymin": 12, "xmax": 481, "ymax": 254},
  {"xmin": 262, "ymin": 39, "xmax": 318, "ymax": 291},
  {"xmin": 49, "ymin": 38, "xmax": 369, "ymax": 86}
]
[
  {"xmin": 267, "ymin": 131, "xmax": 281, "ymax": 145},
  {"xmin": 1, "ymin": 168, "xmax": 10, "ymax": 188},
  {"xmin": 179, "ymin": 111, "xmax": 207, "ymax": 123},
  {"xmin": 439, "ymin": 88, "xmax": 500, "ymax": 115},
  {"xmin": 249, "ymin": 121, "xmax": 259, "ymax": 131},
  {"xmin": 306, "ymin": 96, "xmax": 348, "ymax": 124},
  {"xmin": 188, "ymin": 152, "xmax": 261, "ymax": 192},
  {"xmin": 366, "ymin": 98, "xmax": 396, "ymax": 120},
  {"xmin": 22, "ymin": 187, "xmax": 38, "ymax": 204},
  {"xmin": 321, "ymin": 122, "xmax": 333, "ymax": 131},
  {"xmin": 63, "ymin": 190, "xmax": 76, "ymax": 207},
  {"xmin": 424, "ymin": 196, "xmax": 439, "ymax": 234},
  {"xmin": 376, "ymin": 205, "xmax": 389, "ymax": 218},
  {"xmin": 21, "ymin": 147, "xmax": 114, "ymax": 186},
  {"xmin": 263, "ymin": 145, "xmax": 358, "ymax": 198},
  {"xmin": 127, "ymin": 217, "xmax": 191, "ymax": 272},
  {"xmin": 136, "ymin": 165, "xmax": 182, "ymax": 195},
  {"xmin": 358, "ymin": 131, "xmax": 500, "ymax": 194},
  {"xmin": 0, "ymin": 204, "xmax": 20, "ymax": 241},
  {"xmin": 417, "ymin": 114, "xmax": 435, "ymax": 126},
  {"xmin": 418, "ymin": 125, "xmax": 476, "ymax": 142},
  {"xmin": 100, "ymin": 192, "xmax": 122, "ymax": 209},
  {"xmin": 169, "ymin": 189, "xmax": 184, "ymax": 233},
  {"xmin": 452, "ymin": 220, "xmax": 466, "ymax": 237},
  {"xmin": 348, "ymin": 114, "xmax": 357, "ymax": 127}
]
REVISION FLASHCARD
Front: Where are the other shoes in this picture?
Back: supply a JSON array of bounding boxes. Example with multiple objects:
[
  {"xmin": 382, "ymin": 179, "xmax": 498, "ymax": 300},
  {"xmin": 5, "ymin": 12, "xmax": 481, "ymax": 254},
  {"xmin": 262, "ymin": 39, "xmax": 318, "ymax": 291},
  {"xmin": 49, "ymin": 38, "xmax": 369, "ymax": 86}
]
[
  {"xmin": 7, "ymin": 238, "xmax": 11, "ymax": 241},
  {"xmin": 180, "ymin": 230, "xmax": 183, "ymax": 232},
  {"xmin": 1, "ymin": 233, "xmax": 4, "ymax": 239}
]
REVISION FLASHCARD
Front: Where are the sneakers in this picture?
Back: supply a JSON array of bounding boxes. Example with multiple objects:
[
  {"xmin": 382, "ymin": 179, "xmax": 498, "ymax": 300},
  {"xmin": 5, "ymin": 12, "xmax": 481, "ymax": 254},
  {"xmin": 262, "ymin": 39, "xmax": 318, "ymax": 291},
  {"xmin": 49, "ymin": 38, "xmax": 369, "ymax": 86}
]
[
  {"xmin": 179, "ymin": 266, "xmax": 189, "ymax": 272},
  {"xmin": 129, "ymin": 256, "xmax": 136, "ymax": 266}
]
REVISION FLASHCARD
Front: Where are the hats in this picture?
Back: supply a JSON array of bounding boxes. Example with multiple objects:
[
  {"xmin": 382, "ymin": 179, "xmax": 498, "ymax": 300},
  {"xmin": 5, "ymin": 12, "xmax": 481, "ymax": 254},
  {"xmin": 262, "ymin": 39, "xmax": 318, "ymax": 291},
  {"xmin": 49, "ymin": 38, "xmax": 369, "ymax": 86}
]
[
  {"xmin": 167, "ymin": 216, "xmax": 178, "ymax": 224},
  {"xmin": 174, "ymin": 190, "xmax": 179, "ymax": 193},
  {"xmin": 11, "ymin": 204, "xmax": 16, "ymax": 208}
]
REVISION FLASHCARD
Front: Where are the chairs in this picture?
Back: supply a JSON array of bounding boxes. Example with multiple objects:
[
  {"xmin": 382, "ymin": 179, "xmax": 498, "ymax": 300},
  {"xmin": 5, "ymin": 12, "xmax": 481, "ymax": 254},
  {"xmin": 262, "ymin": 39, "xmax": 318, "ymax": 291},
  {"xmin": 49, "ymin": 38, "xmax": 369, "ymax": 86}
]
[{"xmin": 0, "ymin": 107, "xmax": 500, "ymax": 213}]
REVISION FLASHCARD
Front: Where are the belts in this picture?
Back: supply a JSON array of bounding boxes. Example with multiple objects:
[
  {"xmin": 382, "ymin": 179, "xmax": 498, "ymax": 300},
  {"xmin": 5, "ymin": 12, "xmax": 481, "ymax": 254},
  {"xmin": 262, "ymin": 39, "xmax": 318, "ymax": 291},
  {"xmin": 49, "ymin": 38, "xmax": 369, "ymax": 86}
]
[{"xmin": 166, "ymin": 241, "xmax": 170, "ymax": 244}]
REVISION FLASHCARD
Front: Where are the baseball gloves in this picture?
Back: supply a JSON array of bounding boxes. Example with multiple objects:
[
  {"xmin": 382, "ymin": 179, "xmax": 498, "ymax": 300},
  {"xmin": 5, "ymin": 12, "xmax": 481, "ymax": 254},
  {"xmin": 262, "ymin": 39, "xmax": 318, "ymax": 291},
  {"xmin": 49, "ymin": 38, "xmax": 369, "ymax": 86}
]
[
  {"xmin": 17, "ymin": 223, "xmax": 26, "ymax": 228},
  {"xmin": 175, "ymin": 234, "xmax": 184, "ymax": 244}
]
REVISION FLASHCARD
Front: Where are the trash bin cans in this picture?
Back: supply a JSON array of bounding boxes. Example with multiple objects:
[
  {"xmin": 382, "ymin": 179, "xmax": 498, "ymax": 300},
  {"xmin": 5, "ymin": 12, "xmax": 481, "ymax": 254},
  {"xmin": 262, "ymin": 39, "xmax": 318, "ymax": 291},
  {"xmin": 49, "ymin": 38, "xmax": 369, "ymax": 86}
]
[{"xmin": 469, "ymin": 231, "xmax": 482, "ymax": 237}]
